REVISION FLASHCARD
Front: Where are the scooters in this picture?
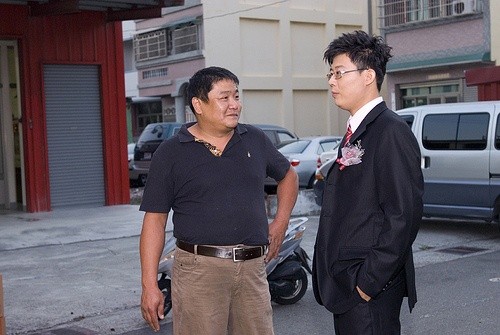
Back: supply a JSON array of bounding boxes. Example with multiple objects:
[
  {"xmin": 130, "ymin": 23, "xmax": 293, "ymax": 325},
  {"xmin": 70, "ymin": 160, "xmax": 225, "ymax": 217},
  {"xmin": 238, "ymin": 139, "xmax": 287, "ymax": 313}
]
[{"xmin": 156, "ymin": 216, "xmax": 312, "ymax": 316}]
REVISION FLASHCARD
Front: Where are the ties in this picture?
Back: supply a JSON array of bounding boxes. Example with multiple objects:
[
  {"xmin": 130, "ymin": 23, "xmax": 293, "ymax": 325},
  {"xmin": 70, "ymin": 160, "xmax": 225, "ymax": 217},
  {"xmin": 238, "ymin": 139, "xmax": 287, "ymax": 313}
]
[{"xmin": 344, "ymin": 124, "xmax": 352, "ymax": 147}]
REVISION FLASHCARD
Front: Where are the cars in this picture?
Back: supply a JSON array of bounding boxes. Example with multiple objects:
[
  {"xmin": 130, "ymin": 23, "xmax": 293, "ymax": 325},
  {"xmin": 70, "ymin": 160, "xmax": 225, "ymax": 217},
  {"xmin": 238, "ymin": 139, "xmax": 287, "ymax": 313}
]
[
  {"xmin": 126, "ymin": 143, "xmax": 149, "ymax": 186},
  {"xmin": 264, "ymin": 136, "xmax": 344, "ymax": 189}
]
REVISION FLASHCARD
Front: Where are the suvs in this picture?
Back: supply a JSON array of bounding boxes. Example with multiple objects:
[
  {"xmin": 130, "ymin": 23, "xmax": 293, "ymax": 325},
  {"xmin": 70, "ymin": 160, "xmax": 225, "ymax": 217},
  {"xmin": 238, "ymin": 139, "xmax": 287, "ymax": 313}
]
[
  {"xmin": 251, "ymin": 123, "xmax": 299, "ymax": 147},
  {"xmin": 132, "ymin": 122, "xmax": 193, "ymax": 173}
]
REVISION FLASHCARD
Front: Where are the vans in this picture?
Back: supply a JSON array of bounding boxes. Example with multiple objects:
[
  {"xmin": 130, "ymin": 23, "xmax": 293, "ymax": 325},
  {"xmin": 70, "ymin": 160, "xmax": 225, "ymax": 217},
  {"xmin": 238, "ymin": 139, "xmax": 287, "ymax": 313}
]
[{"xmin": 311, "ymin": 99, "xmax": 500, "ymax": 224}]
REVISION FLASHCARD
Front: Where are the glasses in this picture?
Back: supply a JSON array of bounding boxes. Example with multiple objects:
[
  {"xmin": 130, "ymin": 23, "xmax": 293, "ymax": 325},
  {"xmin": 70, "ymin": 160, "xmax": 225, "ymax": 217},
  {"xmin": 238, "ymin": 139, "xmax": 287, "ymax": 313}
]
[{"xmin": 326, "ymin": 67, "xmax": 371, "ymax": 82}]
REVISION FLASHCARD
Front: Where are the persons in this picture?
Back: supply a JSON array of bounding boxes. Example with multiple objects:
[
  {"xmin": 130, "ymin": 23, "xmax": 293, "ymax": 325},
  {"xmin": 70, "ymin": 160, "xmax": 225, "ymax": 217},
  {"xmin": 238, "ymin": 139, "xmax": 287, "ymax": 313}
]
[
  {"xmin": 139, "ymin": 66, "xmax": 299, "ymax": 335},
  {"xmin": 312, "ymin": 30, "xmax": 425, "ymax": 335}
]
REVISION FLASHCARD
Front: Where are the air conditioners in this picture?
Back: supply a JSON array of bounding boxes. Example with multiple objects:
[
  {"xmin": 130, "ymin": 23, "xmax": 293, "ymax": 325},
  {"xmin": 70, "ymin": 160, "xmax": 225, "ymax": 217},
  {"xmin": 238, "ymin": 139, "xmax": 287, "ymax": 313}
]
[{"xmin": 453, "ymin": 0, "xmax": 474, "ymax": 16}]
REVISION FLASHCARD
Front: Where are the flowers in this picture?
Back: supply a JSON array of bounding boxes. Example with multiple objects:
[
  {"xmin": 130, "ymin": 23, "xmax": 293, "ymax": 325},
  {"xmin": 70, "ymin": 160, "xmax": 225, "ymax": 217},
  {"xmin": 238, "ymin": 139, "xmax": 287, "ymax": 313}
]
[{"xmin": 336, "ymin": 140, "xmax": 365, "ymax": 171}]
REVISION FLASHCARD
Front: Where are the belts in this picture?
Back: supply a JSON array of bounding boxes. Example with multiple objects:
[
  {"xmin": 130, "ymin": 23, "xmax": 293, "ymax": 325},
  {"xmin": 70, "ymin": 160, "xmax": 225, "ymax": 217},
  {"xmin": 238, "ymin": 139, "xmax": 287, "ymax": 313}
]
[{"xmin": 175, "ymin": 238, "xmax": 267, "ymax": 262}]
